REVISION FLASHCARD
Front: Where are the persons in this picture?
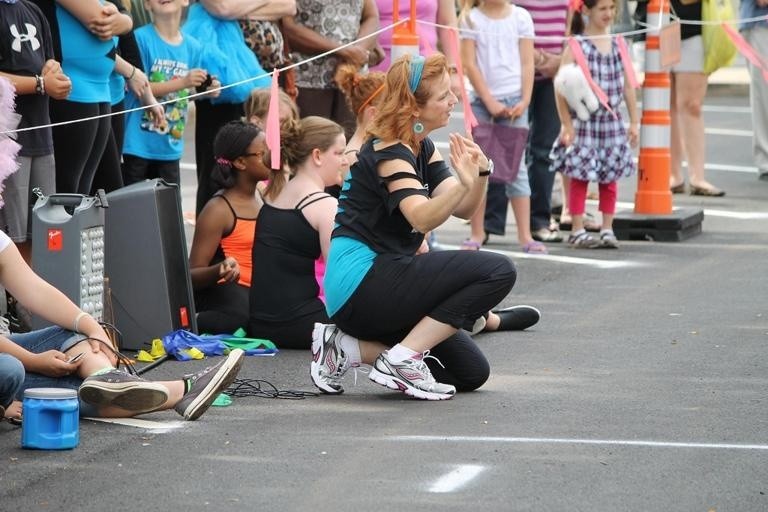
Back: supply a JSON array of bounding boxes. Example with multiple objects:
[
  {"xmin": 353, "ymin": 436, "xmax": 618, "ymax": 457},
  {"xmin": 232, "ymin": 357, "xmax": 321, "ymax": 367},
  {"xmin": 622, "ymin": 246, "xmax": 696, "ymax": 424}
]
[
  {"xmin": 0, "ymin": 230, "xmax": 245, "ymax": 426},
  {"xmin": 309, "ymin": 55, "xmax": 518, "ymax": 399},
  {"xmin": 187, "ymin": 66, "xmax": 540, "ymax": 351},
  {"xmin": 457, "ymin": 1, "xmax": 767, "ymax": 254},
  {"xmin": 2, "ymin": 0, "xmax": 461, "ymax": 333}
]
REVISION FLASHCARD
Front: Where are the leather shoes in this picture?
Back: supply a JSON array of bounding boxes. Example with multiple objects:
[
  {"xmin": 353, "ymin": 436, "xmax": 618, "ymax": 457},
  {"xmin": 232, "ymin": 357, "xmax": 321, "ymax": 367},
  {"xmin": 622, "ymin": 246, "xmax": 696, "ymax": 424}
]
[
  {"xmin": 689, "ymin": 183, "xmax": 725, "ymax": 196},
  {"xmin": 671, "ymin": 178, "xmax": 684, "ymax": 193}
]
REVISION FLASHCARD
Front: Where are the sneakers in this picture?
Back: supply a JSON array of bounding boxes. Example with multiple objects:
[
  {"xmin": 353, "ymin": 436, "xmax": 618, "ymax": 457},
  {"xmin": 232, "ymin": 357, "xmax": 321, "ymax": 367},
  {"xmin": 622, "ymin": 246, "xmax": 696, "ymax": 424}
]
[
  {"xmin": 173, "ymin": 348, "xmax": 245, "ymax": 421},
  {"xmin": 310, "ymin": 321, "xmax": 349, "ymax": 394},
  {"xmin": 78, "ymin": 368, "xmax": 169, "ymax": 413},
  {"xmin": 531, "ymin": 204, "xmax": 619, "ymax": 248},
  {"xmin": 367, "ymin": 349, "xmax": 456, "ymax": 401},
  {"xmin": 492, "ymin": 305, "xmax": 541, "ymax": 331}
]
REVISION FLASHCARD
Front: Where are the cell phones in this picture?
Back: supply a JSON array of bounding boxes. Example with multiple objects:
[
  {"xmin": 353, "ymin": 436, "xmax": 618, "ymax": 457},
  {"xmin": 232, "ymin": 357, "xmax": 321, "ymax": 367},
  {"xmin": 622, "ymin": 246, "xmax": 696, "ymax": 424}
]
[{"xmin": 66, "ymin": 352, "xmax": 84, "ymax": 363}]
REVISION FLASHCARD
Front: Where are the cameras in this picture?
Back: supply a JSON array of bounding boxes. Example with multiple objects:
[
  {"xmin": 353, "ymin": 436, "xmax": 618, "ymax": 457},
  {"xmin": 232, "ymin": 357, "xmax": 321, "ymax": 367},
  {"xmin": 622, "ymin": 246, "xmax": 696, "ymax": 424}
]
[{"xmin": 196, "ymin": 73, "xmax": 213, "ymax": 95}]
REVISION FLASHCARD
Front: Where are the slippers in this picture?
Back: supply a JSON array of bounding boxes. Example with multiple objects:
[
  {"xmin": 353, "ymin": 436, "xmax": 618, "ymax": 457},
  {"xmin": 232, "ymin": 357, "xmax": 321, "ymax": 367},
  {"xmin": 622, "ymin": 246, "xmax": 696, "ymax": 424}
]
[
  {"xmin": 461, "ymin": 240, "xmax": 481, "ymax": 251},
  {"xmin": 523, "ymin": 240, "xmax": 548, "ymax": 254}
]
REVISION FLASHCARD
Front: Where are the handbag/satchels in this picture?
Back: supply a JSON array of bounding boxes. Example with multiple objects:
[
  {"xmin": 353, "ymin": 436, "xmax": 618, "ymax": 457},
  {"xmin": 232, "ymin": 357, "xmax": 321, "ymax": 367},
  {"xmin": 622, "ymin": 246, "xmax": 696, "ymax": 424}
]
[{"xmin": 470, "ymin": 114, "xmax": 530, "ymax": 183}]
